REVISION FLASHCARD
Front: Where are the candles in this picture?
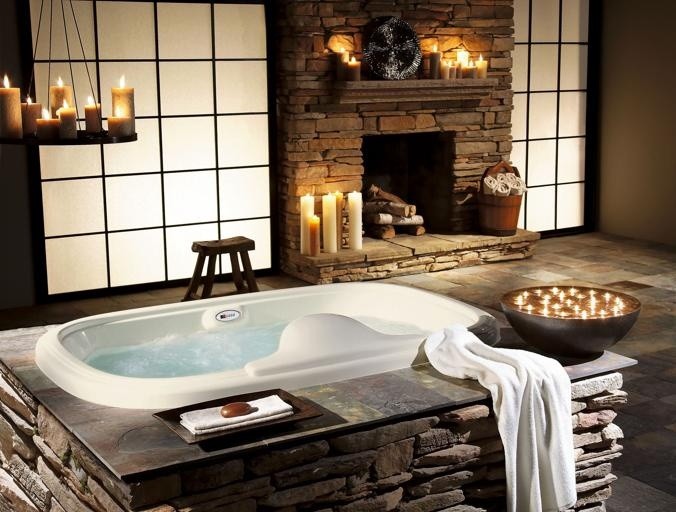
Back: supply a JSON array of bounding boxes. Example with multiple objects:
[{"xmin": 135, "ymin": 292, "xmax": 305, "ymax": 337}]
[
  {"xmin": 0, "ymin": 73, "xmax": 135, "ymax": 138},
  {"xmin": 300, "ymin": 191, "xmax": 363, "ymax": 256},
  {"xmin": 337, "ymin": 47, "xmax": 361, "ymax": 81},
  {"xmin": 430, "ymin": 45, "xmax": 489, "ymax": 78}
]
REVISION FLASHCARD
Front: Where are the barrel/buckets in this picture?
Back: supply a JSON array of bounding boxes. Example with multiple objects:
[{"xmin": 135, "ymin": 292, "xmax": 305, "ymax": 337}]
[{"xmin": 475, "ymin": 161, "xmax": 523, "ymax": 236}]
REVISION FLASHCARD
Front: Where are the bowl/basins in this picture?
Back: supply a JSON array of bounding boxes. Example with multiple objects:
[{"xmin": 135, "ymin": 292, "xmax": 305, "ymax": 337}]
[{"xmin": 500, "ymin": 285, "xmax": 641, "ymax": 357}]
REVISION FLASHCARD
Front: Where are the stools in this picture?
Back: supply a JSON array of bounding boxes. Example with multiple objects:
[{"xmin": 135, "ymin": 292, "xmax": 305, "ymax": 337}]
[{"xmin": 182, "ymin": 236, "xmax": 259, "ymax": 301}]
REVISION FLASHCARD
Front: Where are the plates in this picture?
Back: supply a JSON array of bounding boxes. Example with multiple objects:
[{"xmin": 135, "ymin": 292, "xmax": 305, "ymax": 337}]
[
  {"xmin": 152, "ymin": 389, "xmax": 324, "ymax": 446},
  {"xmin": 361, "ymin": 15, "xmax": 424, "ymax": 81}
]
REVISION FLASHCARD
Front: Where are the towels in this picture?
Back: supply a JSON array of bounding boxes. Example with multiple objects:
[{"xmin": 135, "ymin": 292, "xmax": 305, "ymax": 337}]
[
  {"xmin": 484, "ymin": 173, "xmax": 528, "ymax": 196},
  {"xmin": 179, "ymin": 394, "xmax": 295, "ymax": 435},
  {"xmin": 423, "ymin": 322, "xmax": 579, "ymax": 511}
]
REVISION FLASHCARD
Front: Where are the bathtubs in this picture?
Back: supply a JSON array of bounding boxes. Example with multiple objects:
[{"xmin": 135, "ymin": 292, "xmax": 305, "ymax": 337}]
[{"xmin": 36, "ymin": 282, "xmax": 501, "ymax": 409}]
